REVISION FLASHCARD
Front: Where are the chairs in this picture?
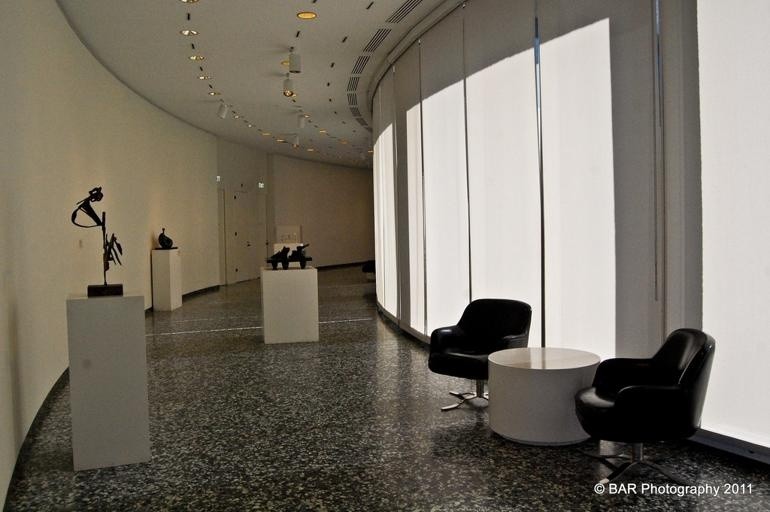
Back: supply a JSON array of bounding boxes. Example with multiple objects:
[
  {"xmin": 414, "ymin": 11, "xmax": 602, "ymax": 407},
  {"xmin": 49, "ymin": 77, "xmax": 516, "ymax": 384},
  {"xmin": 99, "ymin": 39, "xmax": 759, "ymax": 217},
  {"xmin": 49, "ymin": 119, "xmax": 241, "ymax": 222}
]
[
  {"xmin": 427, "ymin": 297, "xmax": 534, "ymax": 414},
  {"xmin": 574, "ymin": 327, "xmax": 716, "ymax": 490}
]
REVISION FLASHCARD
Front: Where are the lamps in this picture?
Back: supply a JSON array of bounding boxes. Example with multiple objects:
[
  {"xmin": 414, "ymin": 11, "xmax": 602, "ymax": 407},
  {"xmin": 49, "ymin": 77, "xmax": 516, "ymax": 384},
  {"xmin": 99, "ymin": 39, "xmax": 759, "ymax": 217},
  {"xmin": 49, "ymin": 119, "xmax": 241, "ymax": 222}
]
[
  {"xmin": 283, "ymin": 50, "xmax": 303, "ymax": 101},
  {"xmin": 296, "ymin": 114, "xmax": 307, "ymax": 132},
  {"xmin": 216, "ymin": 104, "xmax": 228, "ymax": 121}
]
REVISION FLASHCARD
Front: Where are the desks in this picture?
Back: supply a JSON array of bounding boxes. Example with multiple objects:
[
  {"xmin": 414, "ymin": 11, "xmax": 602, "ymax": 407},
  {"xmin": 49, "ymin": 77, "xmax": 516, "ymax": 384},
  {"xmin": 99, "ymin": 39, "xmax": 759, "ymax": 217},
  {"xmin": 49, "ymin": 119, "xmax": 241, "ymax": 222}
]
[{"xmin": 486, "ymin": 345, "xmax": 599, "ymax": 449}]
